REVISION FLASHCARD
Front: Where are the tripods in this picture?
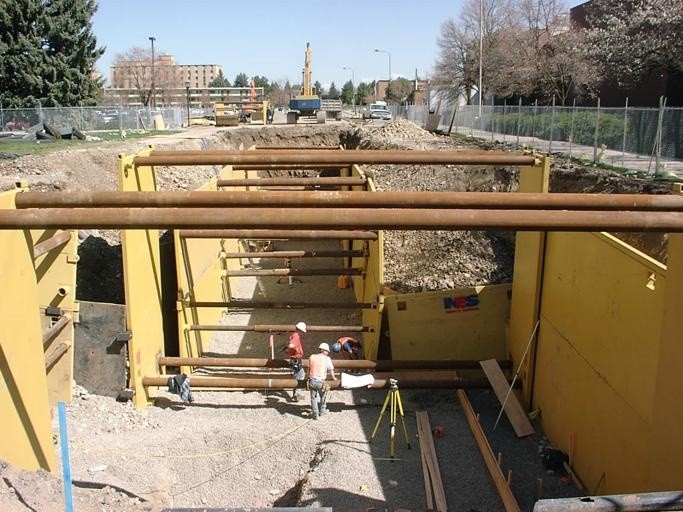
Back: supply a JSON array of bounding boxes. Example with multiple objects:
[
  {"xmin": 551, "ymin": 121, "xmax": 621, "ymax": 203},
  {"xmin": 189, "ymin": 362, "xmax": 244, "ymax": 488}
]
[
  {"xmin": 369, "ymin": 389, "xmax": 412, "ymax": 462},
  {"xmin": 277, "ymin": 276, "xmax": 303, "ymax": 288}
]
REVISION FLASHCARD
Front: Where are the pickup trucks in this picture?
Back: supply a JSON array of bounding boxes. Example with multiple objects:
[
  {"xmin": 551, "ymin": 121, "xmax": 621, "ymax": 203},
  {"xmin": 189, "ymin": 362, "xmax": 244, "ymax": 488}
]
[{"xmin": 362, "ymin": 104, "xmax": 392, "ymax": 119}]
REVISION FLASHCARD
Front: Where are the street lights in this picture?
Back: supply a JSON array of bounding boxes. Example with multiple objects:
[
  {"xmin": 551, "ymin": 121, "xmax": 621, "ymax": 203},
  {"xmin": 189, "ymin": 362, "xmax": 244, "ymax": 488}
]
[
  {"xmin": 342, "ymin": 66, "xmax": 354, "ymax": 100},
  {"xmin": 374, "ymin": 49, "xmax": 392, "ymax": 84},
  {"xmin": 148, "ymin": 37, "xmax": 156, "ymax": 108}
]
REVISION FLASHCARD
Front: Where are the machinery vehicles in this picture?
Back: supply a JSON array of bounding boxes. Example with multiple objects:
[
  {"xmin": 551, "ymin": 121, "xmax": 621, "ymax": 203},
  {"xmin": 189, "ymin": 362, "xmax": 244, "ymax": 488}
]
[
  {"xmin": 286, "ymin": 41, "xmax": 326, "ymax": 124},
  {"xmin": 212, "ymin": 76, "xmax": 274, "ymax": 126}
]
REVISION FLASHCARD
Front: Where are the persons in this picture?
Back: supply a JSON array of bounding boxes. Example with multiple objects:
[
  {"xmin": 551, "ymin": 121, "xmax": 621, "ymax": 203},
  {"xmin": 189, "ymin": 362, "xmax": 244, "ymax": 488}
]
[
  {"xmin": 306, "ymin": 342, "xmax": 337, "ymax": 420},
  {"xmin": 287, "ymin": 321, "xmax": 307, "ymax": 402},
  {"xmin": 332, "ymin": 336, "xmax": 361, "ymax": 373},
  {"xmin": 266, "ymin": 106, "xmax": 274, "ymax": 124}
]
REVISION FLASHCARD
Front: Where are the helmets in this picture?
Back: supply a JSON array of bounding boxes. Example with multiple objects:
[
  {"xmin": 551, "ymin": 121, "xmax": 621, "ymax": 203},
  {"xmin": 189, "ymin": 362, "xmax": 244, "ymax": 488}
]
[
  {"xmin": 332, "ymin": 342, "xmax": 339, "ymax": 353},
  {"xmin": 296, "ymin": 322, "xmax": 307, "ymax": 333},
  {"xmin": 319, "ymin": 342, "xmax": 330, "ymax": 351}
]
